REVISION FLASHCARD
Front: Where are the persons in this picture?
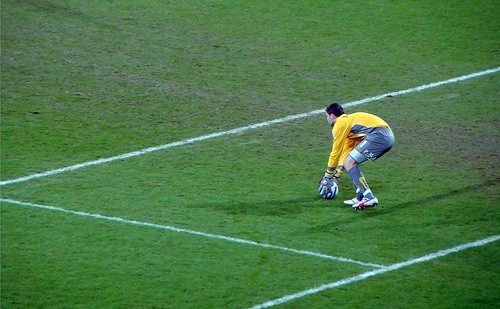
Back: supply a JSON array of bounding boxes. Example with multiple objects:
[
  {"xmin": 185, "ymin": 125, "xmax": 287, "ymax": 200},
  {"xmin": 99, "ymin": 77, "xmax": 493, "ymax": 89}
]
[{"xmin": 318, "ymin": 103, "xmax": 395, "ymax": 210}]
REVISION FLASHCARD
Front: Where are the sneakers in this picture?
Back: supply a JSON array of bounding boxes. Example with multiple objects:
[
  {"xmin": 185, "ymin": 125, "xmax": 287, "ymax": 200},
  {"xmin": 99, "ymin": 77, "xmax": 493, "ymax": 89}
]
[
  {"xmin": 343, "ymin": 198, "xmax": 361, "ymax": 206},
  {"xmin": 352, "ymin": 196, "xmax": 379, "ymax": 209}
]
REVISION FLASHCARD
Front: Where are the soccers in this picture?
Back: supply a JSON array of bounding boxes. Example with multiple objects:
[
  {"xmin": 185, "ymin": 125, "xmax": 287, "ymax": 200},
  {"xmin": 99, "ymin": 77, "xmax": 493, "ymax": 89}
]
[{"xmin": 319, "ymin": 181, "xmax": 338, "ymax": 198}]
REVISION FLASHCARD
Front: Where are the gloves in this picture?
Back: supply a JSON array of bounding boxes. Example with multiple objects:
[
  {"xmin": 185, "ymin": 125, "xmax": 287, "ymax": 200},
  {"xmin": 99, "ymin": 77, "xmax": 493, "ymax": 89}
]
[
  {"xmin": 319, "ymin": 173, "xmax": 333, "ymax": 195},
  {"xmin": 331, "ymin": 172, "xmax": 341, "ymax": 184}
]
